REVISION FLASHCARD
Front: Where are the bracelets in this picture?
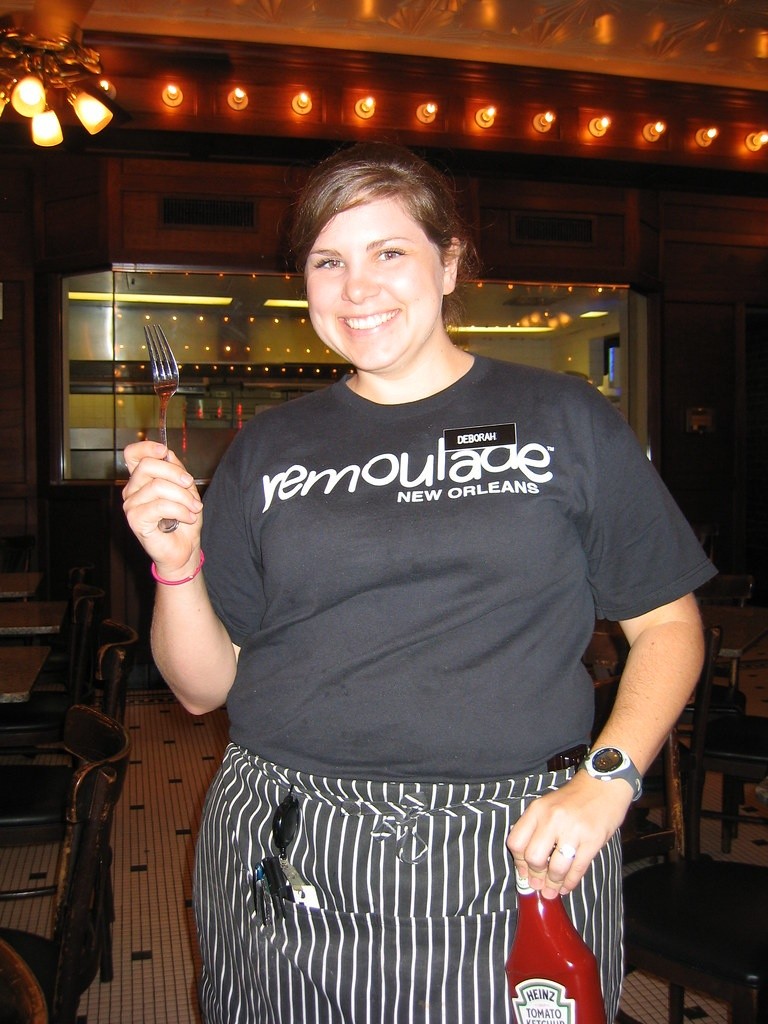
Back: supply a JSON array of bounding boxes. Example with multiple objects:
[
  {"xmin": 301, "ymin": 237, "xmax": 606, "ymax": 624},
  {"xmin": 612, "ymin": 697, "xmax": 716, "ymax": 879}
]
[{"xmin": 151, "ymin": 548, "xmax": 206, "ymax": 585}]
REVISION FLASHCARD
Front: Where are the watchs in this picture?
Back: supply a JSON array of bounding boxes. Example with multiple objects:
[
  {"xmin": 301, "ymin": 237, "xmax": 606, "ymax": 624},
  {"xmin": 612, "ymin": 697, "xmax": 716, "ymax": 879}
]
[{"xmin": 577, "ymin": 746, "xmax": 643, "ymax": 800}]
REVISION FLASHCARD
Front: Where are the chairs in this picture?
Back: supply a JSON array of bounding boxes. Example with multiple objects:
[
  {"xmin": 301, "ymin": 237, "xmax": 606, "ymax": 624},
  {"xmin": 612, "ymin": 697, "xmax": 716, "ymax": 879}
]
[
  {"xmin": 0, "ymin": 559, "xmax": 138, "ymax": 1024},
  {"xmin": 546, "ymin": 516, "xmax": 768, "ymax": 1024}
]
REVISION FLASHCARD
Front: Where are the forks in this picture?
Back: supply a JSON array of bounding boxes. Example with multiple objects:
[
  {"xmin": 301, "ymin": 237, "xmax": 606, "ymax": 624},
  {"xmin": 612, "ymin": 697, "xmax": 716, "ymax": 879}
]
[{"xmin": 144, "ymin": 322, "xmax": 180, "ymax": 532}]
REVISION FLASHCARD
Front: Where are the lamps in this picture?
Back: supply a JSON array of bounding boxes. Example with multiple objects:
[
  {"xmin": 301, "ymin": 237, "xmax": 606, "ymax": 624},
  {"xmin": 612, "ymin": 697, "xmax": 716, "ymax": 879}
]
[{"xmin": 0, "ymin": 11, "xmax": 119, "ymax": 147}]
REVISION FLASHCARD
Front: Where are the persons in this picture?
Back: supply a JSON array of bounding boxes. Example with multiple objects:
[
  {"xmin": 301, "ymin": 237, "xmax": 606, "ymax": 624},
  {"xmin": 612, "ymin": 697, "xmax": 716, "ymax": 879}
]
[{"xmin": 122, "ymin": 141, "xmax": 720, "ymax": 1023}]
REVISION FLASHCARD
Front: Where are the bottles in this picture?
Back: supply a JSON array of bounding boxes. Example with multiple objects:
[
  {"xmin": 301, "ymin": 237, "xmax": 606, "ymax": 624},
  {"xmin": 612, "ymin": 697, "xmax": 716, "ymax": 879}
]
[{"xmin": 503, "ymin": 827, "xmax": 608, "ymax": 1024}]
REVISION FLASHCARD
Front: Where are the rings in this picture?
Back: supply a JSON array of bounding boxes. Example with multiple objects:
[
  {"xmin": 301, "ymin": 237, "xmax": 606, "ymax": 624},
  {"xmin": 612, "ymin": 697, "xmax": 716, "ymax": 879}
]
[{"xmin": 556, "ymin": 845, "xmax": 576, "ymax": 858}]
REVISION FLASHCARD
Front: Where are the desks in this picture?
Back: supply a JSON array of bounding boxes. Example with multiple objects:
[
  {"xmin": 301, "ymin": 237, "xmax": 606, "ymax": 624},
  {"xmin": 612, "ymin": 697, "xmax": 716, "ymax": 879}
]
[
  {"xmin": 1, "ymin": 571, "xmax": 44, "ymax": 598},
  {"xmin": 702, "ymin": 604, "xmax": 768, "ymax": 700},
  {"xmin": 0, "ymin": 640, "xmax": 50, "ymax": 702},
  {"xmin": 1, "ymin": 596, "xmax": 66, "ymax": 640}
]
